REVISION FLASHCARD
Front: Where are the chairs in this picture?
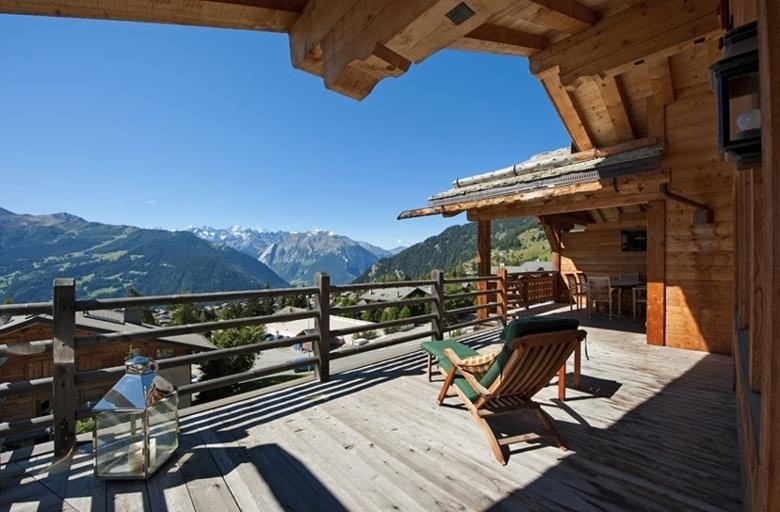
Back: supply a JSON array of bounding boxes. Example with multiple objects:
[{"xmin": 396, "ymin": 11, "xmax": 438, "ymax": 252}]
[
  {"xmin": 565, "ymin": 273, "xmax": 647, "ymax": 322},
  {"xmin": 420, "ymin": 315, "xmax": 585, "ymax": 467}
]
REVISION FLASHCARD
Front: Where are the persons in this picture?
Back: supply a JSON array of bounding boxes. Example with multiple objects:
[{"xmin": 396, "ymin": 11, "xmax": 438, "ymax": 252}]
[{"xmin": 274, "ymin": 331, "xmax": 279, "ymax": 339}]
